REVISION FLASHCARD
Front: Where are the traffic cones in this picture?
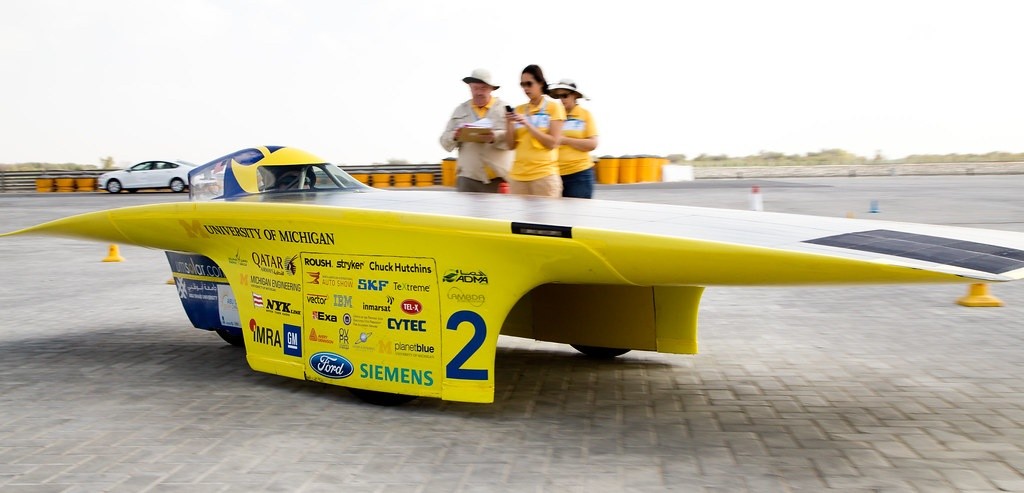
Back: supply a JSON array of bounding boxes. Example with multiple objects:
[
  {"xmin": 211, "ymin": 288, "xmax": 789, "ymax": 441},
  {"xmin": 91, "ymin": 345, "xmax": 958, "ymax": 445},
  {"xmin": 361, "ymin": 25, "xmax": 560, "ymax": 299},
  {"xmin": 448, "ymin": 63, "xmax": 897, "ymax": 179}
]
[
  {"xmin": 956, "ymin": 281, "xmax": 1004, "ymax": 306},
  {"xmin": 100, "ymin": 245, "xmax": 123, "ymax": 264},
  {"xmin": 745, "ymin": 183, "xmax": 765, "ymax": 211},
  {"xmin": 168, "ymin": 278, "xmax": 179, "ymax": 288}
]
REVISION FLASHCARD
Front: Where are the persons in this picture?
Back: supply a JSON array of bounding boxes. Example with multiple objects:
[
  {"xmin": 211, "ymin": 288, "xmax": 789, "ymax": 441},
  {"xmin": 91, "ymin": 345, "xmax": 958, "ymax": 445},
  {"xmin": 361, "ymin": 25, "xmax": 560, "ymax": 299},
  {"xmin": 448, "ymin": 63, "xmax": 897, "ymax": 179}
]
[
  {"xmin": 505, "ymin": 65, "xmax": 563, "ymax": 198},
  {"xmin": 549, "ymin": 80, "xmax": 599, "ymax": 199},
  {"xmin": 439, "ymin": 68, "xmax": 515, "ymax": 193}
]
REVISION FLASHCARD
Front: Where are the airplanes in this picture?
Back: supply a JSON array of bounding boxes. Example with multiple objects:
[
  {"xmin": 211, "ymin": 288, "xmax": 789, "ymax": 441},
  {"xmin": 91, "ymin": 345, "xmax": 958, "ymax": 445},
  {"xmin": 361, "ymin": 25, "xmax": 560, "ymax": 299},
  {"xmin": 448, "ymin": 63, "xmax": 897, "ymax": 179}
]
[{"xmin": 0, "ymin": 143, "xmax": 1024, "ymax": 409}]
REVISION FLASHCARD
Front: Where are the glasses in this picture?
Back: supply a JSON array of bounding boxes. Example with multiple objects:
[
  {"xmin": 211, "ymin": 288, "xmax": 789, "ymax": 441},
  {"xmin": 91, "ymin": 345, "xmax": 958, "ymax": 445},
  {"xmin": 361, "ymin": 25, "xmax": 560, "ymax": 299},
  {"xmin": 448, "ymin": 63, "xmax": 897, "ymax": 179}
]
[
  {"xmin": 520, "ymin": 81, "xmax": 537, "ymax": 87},
  {"xmin": 279, "ymin": 175, "xmax": 311, "ymax": 186},
  {"xmin": 550, "ymin": 93, "xmax": 570, "ymax": 99}
]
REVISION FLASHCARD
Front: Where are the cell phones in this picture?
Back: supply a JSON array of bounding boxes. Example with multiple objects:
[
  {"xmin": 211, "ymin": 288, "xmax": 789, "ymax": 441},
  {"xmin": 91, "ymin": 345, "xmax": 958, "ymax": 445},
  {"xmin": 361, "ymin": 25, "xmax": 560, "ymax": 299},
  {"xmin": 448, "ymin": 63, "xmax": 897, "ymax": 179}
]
[{"xmin": 505, "ymin": 105, "xmax": 513, "ymax": 113}]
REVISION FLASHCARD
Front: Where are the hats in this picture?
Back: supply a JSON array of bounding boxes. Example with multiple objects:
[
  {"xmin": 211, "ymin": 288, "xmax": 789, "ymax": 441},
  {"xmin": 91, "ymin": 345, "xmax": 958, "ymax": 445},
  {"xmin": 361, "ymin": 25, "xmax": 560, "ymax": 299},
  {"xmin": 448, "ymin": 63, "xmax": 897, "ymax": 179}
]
[
  {"xmin": 462, "ymin": 68, "xmax": 500, "ymax": 90},
  {"xmin": 548, "ymin": 77, "xmax": 583, "ymax": 98}
]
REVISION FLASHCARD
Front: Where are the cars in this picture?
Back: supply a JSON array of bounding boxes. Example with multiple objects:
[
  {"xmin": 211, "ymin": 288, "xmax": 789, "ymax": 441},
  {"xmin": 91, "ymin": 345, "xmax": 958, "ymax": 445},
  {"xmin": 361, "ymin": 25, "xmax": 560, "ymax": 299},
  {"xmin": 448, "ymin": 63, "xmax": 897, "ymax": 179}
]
[{"xmin": 96, "ymin": 157, "xmax": 205, "ymax": 192}]
